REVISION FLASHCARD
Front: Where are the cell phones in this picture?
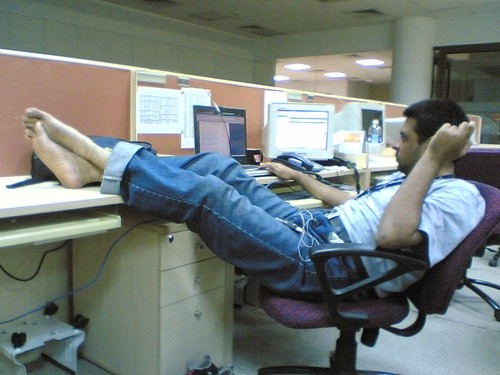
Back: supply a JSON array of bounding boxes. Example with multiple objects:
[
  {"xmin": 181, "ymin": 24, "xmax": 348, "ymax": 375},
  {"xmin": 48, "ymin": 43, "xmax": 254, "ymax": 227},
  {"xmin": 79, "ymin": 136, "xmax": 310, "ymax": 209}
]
[{"xmin": 276, "ymin": 217, "xmax": 303, "ymax": 233}]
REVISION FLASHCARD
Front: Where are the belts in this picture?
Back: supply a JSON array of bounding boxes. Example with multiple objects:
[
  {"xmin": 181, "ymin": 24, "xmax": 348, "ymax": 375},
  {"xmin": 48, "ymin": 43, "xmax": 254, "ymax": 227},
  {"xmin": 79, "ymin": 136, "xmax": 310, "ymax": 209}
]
[{"xmin": 323, "ymin": 211, "xmax": 379, "ymax": 301}]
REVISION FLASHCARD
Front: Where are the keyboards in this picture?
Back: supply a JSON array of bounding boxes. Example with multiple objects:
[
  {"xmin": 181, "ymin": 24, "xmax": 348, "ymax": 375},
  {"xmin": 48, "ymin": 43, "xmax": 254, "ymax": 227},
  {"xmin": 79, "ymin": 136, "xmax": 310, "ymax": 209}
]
[
  {"xmin": 311, "ymin": 159, "xmax": 345, "ymax": 166},
  {"xmin": 242, "ymin": 163, "xmax": 272, "ymax": 176}
]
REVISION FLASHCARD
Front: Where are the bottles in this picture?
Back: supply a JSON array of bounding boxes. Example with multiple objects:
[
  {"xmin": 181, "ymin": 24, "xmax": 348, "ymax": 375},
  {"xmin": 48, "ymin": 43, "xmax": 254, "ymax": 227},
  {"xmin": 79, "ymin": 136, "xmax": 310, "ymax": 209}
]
[{"xmin": 366, "ymin": 118, "xmax": 382, "ymax": 162}]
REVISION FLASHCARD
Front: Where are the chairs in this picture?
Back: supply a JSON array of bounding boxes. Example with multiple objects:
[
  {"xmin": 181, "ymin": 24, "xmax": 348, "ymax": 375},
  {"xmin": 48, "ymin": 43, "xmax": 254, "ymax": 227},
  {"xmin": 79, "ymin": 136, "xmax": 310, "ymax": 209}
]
[{"xmin": 244, "ymin": 180, "xmax": 499, "ymax": 375}]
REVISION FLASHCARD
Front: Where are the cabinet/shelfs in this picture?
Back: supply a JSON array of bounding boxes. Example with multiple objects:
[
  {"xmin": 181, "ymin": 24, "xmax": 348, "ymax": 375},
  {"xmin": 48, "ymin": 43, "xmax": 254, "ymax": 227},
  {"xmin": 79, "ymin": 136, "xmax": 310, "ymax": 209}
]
[{"xmin": 72, "ymin": 221, "xmax": 232, "ymax": 375}]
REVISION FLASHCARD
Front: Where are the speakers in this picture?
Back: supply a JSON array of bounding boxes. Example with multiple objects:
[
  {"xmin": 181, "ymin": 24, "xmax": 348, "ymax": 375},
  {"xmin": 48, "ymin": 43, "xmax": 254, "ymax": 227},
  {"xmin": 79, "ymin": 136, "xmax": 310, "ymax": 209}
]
[{"xmin": 246, "ymin": 147, "xmax": 264, "ymax": 165}]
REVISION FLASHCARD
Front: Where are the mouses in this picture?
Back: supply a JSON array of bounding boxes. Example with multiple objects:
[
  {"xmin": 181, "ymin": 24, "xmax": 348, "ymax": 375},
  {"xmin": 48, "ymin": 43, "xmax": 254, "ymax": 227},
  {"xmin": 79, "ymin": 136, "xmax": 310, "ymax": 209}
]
[{"xmin": 258, "ymin": 166, "xmax": 270, "ymax": 171}]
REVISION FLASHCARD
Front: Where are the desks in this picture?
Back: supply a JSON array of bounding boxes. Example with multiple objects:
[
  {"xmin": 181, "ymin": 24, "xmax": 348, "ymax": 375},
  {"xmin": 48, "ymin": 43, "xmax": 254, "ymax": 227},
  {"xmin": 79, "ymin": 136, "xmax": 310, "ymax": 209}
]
[
  {"xmin": 1, "ymin": 49, "xmax": 132, "ymax": 375},
  {"xmin": 131, "ymin": 62, "xmax": 482, "ymax": 374}
]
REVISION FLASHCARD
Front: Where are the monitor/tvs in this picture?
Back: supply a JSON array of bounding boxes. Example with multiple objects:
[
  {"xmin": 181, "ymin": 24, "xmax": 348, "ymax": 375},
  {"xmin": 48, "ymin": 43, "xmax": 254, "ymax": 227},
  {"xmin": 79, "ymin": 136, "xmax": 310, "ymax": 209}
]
[
  {"xmin": 263, "ymin": 102, "xmax": 336, "ymax": 158},
  {"xmin": 336, "ymin": 102, "xmax": 385, "ymax": 150},
  {"xmin": 193, "ymin": 104, "xmax": 246, "ymax": 161}
]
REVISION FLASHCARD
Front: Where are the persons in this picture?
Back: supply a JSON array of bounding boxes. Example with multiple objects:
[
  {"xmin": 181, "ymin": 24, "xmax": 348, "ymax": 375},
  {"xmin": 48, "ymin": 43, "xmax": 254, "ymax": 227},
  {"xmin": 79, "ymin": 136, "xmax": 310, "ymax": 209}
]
[{"xmin": 22, "ymin": 98, "xmax": 486, "ymax": 303}]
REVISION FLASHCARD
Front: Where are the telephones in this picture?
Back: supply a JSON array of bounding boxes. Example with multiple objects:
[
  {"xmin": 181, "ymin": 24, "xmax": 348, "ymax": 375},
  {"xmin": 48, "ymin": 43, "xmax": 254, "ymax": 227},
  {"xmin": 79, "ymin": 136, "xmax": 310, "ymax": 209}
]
[
  {"xmin": 271, "ymin": 153, "xmax": 331, "ymax": 185},
  {"xmin": 334, "ymin": 156, "xmax": 360, "ymax": 194}
]
